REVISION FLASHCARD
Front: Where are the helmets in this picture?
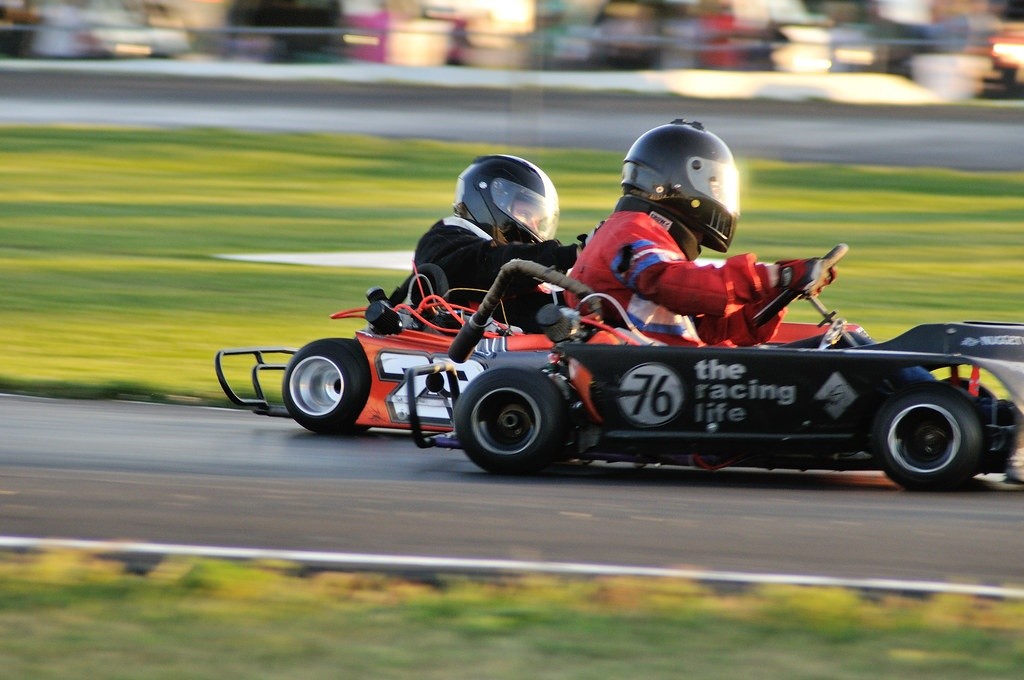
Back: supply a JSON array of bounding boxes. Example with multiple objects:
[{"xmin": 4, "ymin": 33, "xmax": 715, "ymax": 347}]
[
  {"xmin": 452, "ymin": 155, "xmax": 561, "ymax": 245},
  {"xmin": 622, "ymin": 118, "xmax": 742, "ymax": 254}
]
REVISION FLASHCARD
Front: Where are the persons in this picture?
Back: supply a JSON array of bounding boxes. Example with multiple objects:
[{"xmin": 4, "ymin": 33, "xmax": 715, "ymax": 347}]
[
  {"xmin": 562, "ymin": 119, "xmax": 836, "ymax": 349},
  {"xmin": 408, "ymin": 154, "xmax": 605, "ymax": 335}
]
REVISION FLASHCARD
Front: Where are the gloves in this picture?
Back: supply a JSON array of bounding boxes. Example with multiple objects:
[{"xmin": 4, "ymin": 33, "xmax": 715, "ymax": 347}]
[
  {"xmin": 577, "ymin": 219, "xmax": 605, "ymax": 251},
  {"xmin": 780, "ymin": 243, "xmax": 850, "ymax": 298}
]
